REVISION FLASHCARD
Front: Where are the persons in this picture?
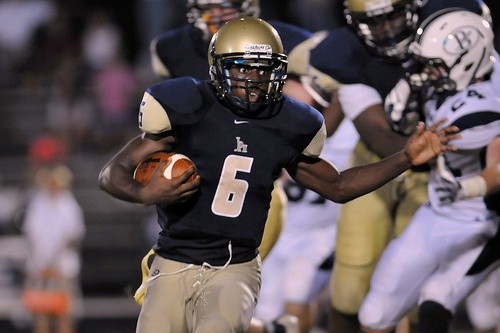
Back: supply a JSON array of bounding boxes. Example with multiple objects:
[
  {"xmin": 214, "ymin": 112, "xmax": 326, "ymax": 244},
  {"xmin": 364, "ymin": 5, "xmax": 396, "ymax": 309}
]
[
  {"xmin": 97, "ymin": 16, "xmax": 462, "ymax": 333},
  {"xmin": 0, "ymin": 0, "xmax": 500, "ymax": 333}
]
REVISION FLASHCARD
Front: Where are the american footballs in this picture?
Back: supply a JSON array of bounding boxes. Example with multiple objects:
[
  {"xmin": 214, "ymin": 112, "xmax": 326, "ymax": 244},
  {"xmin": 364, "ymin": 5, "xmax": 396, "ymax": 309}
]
[{"xmin": 133, "ymin": 152, "xmax": 198, "ymax": 184}]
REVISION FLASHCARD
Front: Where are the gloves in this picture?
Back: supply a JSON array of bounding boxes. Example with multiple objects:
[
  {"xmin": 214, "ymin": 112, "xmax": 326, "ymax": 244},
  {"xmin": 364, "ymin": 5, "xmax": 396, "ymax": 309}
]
[{"xmin": 434, "ymin": 152, "xmax": 463, "ymax": 204}]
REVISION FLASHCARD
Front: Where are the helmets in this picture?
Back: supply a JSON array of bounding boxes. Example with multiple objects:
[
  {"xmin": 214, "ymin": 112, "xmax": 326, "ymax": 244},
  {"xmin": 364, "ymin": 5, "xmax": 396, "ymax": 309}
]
[
  {"xmin": 207, "ymin": 16, "xmax": 285, "ymax": 75},
  {"xmin": 188, "ymin": 0, "xmax": 261, "ymax": 21},
  {"xmin": 345, "ymin": 0, "xmax": 419, "ymax": 26},
  {"xmin": 406, "ymin": 7, "xmax": 496, "ymax": 91}
]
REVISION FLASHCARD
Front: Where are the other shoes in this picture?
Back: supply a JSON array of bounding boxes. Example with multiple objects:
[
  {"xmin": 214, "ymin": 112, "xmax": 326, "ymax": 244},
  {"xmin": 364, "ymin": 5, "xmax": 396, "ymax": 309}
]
[{"xmin": 277, "ymin": 314, "xmax": 300, "ymax": 333}]
